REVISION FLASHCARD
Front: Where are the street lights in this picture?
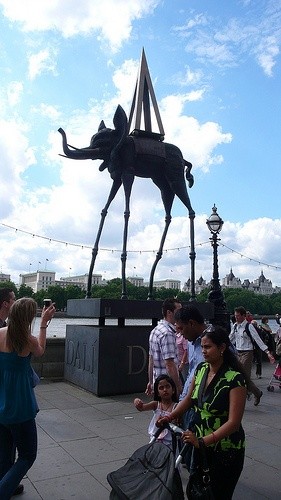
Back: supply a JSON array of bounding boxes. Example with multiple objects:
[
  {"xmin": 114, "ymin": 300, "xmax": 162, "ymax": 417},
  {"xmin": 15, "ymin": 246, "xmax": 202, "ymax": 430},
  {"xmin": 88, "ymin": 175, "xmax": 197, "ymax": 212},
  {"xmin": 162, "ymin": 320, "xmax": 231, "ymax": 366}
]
[{"xmin": 205, "ymin": 202, "xmax": 233, "ymax": 326}]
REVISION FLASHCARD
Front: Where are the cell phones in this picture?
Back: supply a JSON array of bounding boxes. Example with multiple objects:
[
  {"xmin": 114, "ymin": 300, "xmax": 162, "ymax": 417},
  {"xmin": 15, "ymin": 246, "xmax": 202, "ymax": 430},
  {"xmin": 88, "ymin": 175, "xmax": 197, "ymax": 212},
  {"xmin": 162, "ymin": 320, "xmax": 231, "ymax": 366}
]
[{"xmin": 44, "ymin": 299, "xmax": 52, "ymax": 311}]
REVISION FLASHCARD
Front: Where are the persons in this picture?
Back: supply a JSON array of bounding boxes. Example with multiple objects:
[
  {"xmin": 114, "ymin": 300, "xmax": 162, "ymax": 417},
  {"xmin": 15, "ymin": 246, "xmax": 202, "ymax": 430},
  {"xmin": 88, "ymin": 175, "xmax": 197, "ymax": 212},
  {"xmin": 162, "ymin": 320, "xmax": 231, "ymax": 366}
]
[
  {"xmin": 134, "ymin": 298, "xmax": 281, "ymax": 500},
  {"xmin": 0, "ymin": 289, "xmax": 55, "ymax": 500}
]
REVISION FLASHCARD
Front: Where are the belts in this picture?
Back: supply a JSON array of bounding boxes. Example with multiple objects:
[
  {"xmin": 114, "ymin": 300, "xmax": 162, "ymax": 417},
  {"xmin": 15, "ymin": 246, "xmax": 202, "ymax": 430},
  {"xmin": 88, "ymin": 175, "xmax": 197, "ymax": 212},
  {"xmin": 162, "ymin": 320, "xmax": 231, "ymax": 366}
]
[{"xmin": 236, "ymin": 349, "xmax": 252, "ymax": 354}]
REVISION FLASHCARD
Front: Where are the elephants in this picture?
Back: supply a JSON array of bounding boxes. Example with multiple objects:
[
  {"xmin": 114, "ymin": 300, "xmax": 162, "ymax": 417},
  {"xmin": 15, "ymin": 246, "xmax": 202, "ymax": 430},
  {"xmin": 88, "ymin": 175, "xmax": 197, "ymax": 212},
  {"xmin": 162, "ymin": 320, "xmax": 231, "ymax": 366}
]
[{"xmin": 56, "ymin": 102, "xmax": 198, "ymax": 325}]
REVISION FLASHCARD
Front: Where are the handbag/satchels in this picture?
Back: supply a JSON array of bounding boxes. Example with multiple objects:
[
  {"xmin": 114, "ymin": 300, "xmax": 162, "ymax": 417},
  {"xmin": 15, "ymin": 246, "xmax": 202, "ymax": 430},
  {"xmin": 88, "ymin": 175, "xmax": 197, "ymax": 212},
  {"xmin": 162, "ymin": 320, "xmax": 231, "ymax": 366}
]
[{"xmin": 184, "ymin": 435, "xmax": 217, "ymax": 499}]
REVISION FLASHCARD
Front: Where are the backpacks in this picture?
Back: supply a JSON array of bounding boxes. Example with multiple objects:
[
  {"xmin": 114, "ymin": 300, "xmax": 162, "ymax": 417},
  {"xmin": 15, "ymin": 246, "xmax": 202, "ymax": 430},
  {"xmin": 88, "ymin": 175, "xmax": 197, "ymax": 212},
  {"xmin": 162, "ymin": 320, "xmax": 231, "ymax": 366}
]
[{"xmin": 245, "ymin": 322, "xmax": 276, "ymax": 362}]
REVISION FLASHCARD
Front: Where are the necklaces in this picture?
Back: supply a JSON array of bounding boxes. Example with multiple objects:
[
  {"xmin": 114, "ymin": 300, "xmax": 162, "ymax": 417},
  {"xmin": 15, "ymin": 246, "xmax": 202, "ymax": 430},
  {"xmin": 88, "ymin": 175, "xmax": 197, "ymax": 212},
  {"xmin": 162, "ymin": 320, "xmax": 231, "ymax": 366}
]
[{"xmin": 160, "ymin": 401, "xmax": 173, "ymax": 411}]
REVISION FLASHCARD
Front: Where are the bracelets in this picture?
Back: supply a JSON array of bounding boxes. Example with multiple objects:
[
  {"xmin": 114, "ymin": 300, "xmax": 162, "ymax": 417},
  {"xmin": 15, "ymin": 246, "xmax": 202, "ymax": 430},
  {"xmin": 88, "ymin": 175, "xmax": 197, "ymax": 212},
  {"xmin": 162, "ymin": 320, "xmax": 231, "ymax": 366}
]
[
  {"xmin": 182, "ymin": 362, "xmax": 184, "ymax": 364},
  {"xmin": 266, "ymin": 351, "xmax": 270, "ymax": 355},
  {"xmin": 180, "ymin": 361, "xmax": 185, "ymax": 366},
  {"xmin": 198, "ymin": 437, "xmax": 205, "ymax": 449},
  {"xmin": 211, "ymin": 432, "xmax": 218, "ymax": 443},
  {"xmin": 40, "ymin": 326, "xmax": 47, "ymax": 329}
]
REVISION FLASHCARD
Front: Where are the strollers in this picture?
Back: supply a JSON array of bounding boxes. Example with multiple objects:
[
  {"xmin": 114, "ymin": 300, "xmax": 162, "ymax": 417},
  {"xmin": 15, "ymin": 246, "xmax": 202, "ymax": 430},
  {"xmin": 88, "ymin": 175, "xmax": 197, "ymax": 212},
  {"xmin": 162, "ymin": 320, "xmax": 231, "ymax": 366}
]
[{"xmin": 106, "ymin": 422, "xmax": 190, "ymax": 500}]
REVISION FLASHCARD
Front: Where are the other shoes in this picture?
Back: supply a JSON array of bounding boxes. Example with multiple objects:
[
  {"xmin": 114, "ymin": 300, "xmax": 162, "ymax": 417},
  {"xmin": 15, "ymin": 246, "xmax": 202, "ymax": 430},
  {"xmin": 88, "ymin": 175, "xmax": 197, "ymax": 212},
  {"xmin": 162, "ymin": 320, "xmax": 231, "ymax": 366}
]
[
  {"xmin": 246, "ymin": 393, "xmax": 252, "ymax": 402},
  {"xmin": 253, "ymin": 390, "xmax": 263, "ymax": 406},
  {"xmin": 10, "ymin": 482, "xmax": 25, "ymax": 497}
]
